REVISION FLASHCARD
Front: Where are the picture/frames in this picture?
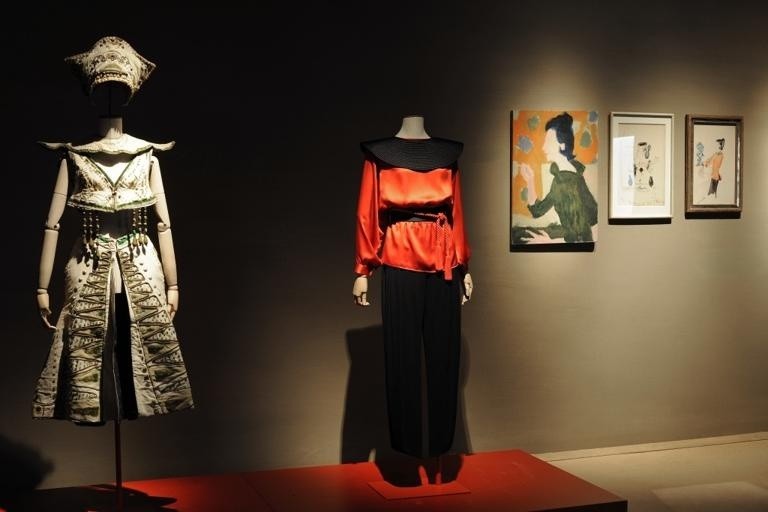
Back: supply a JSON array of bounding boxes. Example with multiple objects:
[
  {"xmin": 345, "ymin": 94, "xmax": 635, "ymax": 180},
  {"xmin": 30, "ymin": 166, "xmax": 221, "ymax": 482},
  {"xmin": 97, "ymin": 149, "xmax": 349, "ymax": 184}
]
[
  {"xmin": 684, "ymin": 112, "xmax": 743, "ymax": 214},
  {"xmin": 610, "ymin": 110, "xmax": 673, "ymax": 221}
]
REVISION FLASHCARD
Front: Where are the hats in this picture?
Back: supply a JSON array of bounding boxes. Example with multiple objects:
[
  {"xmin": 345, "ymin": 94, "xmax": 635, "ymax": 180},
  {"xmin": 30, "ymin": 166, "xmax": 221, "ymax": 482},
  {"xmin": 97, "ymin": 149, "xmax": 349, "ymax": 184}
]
[{"xmin": 62, "ymin": 34, "xmax": 157, "ymax": 103}]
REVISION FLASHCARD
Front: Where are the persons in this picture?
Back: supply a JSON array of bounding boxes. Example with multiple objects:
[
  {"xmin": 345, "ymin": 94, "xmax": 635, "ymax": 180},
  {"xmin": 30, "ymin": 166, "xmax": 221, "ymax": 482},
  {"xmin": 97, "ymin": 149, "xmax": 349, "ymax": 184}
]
[
  {"xmin": 352, "ymin": 116, "xmax": 475, "ymax": 461},
  {"xmin": 703, "ymin": 137, "xmax": 726, "ymax": 198},
  {"xmin": 28, "ymin": 35, "xmax": 195, "ymax": 426},
  {"xmin": 511, "ymin": 113, "xmax": 597, "ymax": 245}
]
